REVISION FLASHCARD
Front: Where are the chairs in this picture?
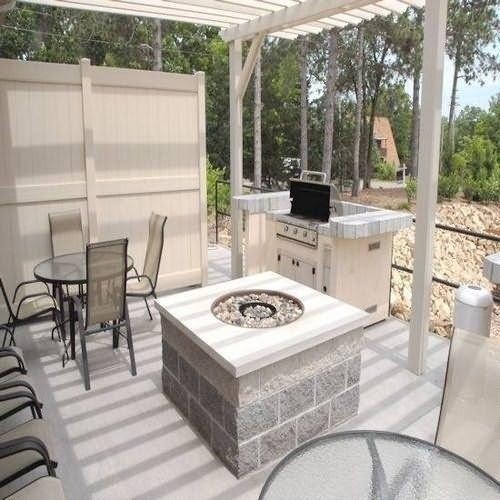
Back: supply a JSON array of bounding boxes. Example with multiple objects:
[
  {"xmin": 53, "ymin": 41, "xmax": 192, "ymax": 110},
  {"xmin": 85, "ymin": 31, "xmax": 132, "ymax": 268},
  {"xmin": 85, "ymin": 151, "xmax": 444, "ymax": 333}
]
[
  {"xmin": 50, "ymin": 208, "xmax": 90, "ymax": 322},
  {"xmin": 69, "ymin": 238, "xmax": 136, "ymax": 390},
  {"xmin": 104, "ymin": 211, "xmax": 167, "ymax": 346},
  {"xmin": 1, "ymin": 274, "xmax": 63, "ymax": 346},
  {"xmin": 1, "ymin": 325, "xmax": 64, "ymax": 500}
]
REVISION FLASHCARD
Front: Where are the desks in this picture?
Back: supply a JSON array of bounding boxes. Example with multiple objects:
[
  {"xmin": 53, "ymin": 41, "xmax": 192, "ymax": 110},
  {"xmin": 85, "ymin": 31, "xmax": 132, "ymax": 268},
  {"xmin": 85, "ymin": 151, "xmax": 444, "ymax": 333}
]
[
  {"xmin": 36, "ymin": 252, "xmax": 135, "ymax": 356},
  {"xmin": 261, "ymin": 432, "xmax": 500, "ymax": 500}
]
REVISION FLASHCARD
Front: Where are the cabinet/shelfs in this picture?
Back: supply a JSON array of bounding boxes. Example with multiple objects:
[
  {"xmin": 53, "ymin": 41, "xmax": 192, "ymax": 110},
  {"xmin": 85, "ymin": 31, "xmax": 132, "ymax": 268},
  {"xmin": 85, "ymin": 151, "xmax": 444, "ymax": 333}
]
[{"xmin": 267, "ymin": 209, "xmax": 393, "ymax": 327}]
